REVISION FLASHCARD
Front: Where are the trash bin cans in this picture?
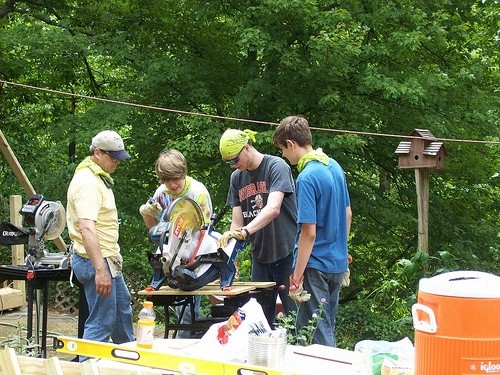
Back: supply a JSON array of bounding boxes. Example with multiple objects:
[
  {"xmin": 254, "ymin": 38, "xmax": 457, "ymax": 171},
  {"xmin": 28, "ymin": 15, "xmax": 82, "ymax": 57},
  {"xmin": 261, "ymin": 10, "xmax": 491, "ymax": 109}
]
[{"xmin": 411, "ymin": 270, "xmax": 499, "ymax": 375}]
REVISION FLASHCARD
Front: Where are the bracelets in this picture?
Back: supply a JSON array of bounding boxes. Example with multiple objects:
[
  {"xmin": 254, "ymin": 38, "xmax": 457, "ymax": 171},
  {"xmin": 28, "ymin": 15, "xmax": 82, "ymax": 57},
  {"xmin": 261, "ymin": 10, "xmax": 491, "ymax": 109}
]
[{"xmin": 241, "ymin": 226, "xmax": 250, "ymax": 238}]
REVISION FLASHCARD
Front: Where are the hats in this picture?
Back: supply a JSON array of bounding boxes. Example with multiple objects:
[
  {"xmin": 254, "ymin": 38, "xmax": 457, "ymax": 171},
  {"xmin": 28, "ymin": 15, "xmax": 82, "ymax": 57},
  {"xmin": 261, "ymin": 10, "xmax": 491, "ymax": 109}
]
[{"xmin": 91, "ymin": 130, "xmax": 132, "ymax": 160}]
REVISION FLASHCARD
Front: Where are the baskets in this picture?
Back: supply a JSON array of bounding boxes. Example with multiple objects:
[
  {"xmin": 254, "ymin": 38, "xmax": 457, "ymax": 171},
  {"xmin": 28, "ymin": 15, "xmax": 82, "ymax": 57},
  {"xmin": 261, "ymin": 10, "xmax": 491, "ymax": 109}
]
[{"xmin": 247, "ymin": 328, "xmax": 287, "ymax": 368}]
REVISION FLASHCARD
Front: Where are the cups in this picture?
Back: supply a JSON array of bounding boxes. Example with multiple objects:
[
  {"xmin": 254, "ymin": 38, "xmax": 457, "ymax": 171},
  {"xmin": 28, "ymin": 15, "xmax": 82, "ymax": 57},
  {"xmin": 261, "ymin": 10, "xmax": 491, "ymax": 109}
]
[{"xmin": 248, "ymin": 337, "xmax": 289, "ymax": 375}]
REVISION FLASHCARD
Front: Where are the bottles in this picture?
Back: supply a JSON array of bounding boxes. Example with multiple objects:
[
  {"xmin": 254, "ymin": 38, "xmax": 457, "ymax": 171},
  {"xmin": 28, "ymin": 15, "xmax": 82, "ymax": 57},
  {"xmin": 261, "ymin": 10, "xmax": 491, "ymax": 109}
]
[{"xmin": 137, "ymin": 302, "xmax": 156, "ymax": 350}]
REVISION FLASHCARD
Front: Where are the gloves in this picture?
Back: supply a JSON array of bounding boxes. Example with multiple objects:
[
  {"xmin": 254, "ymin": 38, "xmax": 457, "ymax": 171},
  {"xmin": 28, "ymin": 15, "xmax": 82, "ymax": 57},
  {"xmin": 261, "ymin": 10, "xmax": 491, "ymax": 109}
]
[
  {"xmin": 340, "ymin": 266, "xmax": 350, "ymax": 287},
  {"xmin": 139, "ymin": 202, "xmax": 163, "ymax": 218},
  {"xmin": 289, "ymin": 273, "xmax": 312, "ymax": 303},
  {"xmin": 216, "ymin": 226, "xmax": 246, "ymax": 249}
]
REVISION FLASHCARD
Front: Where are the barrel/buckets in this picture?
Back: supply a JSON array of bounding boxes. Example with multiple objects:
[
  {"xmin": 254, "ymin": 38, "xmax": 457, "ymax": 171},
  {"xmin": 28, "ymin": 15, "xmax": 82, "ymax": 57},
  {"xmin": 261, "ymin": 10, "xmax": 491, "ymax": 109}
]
[{"xmin": 413, "ymin": 270, "xmax": 500, "ymax": 375}]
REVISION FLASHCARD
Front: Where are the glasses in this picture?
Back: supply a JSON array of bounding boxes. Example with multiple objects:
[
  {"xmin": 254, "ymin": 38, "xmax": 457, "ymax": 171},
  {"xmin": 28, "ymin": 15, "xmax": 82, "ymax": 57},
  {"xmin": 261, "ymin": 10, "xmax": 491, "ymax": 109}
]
[{"xmin": 225, "ymin": 146, "xmax": 245, "ymax": 165}]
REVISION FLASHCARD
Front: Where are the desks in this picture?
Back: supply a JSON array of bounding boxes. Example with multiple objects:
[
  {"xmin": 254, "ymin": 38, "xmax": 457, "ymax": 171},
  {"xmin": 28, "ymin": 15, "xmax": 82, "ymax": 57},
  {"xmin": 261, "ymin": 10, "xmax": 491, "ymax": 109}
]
[
  {"xmin": 93, "ymin": 339, "xmax": 353, "ymax": 375},
  {"xmin": 0, "ymin": 265, "xmax": 88, "ymax": 361},
  {"xmin": 137, "ymin": 281, "xmax": 276, "ymax": 339}
]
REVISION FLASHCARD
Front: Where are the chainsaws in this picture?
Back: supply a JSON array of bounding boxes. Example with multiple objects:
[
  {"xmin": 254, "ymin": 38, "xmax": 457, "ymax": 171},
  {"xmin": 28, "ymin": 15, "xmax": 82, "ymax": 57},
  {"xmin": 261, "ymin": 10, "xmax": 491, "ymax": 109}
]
[
  {"xmin": 145, "ymin": 197, "xmax": 247, "ymax": 291},
  {"xmin": 19, "ymin": 194, "xmax": 70, "ymax": 269}
]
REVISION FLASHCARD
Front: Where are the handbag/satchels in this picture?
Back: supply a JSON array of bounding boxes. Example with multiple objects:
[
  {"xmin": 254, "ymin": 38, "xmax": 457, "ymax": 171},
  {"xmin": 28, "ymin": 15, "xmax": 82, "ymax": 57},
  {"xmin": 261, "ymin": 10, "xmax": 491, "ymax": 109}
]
[{"xmin": 188, "ymin": 298, "xmax": 273, "ymax": 360}]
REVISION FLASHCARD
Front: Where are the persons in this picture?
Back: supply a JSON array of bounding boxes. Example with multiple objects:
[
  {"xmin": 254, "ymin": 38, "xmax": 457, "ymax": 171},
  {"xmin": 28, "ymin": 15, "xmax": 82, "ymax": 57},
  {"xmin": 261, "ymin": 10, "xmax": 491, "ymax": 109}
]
[
  {"xmin": 66, "ymin": 130, "xmax": 136, "ymax": 363},
  {"xmin": 139, "ymin": 149, "xmax": 212, "ymax": 339},
  {"xmin": 273, "ymin": 115, "xmax": 352, "ymax": 347},
  {"xmin": 220, "ymin": 129, "xmax": 299, "ymax": 345}
]
